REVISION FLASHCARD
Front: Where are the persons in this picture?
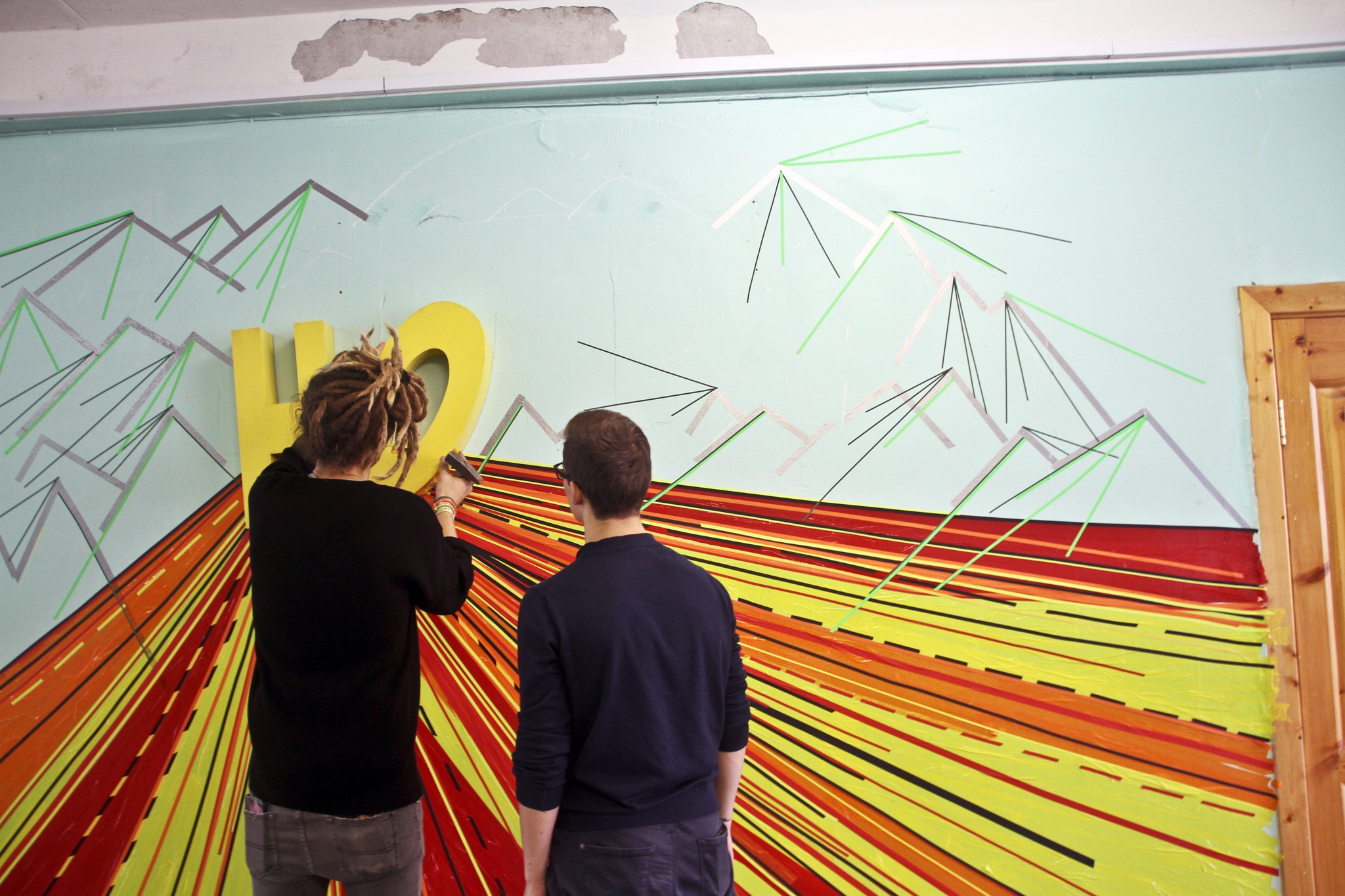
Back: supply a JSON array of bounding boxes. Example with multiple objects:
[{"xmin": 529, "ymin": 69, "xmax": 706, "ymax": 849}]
[
  {"xmin": 512, "ymin": 410, "xmax": 751, "ymax": 896},
  {"xmin": 245, "ymin": 324, "xmax": 475, "ymax": 896}
]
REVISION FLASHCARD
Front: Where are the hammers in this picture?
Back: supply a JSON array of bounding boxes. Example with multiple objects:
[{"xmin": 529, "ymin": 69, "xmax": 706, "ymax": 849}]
[{"xmin": 444, "ymin": 451, "xmax": 483, "ymax": 485}]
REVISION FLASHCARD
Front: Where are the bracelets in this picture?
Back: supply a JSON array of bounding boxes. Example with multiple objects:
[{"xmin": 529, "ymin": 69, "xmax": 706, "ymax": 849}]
[
  {"xmin": 721, "ymin": 818, "xmax": 731, "ymax": 822},
  {"xmin": 430, "ymin": 496, "xmax": 457, "ymax": 520}
]
